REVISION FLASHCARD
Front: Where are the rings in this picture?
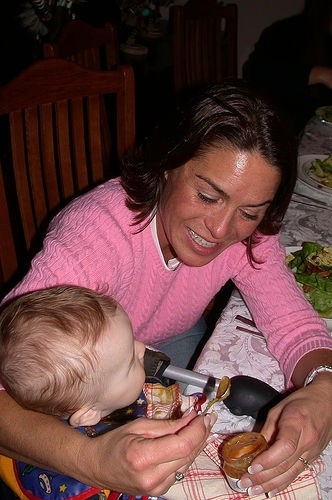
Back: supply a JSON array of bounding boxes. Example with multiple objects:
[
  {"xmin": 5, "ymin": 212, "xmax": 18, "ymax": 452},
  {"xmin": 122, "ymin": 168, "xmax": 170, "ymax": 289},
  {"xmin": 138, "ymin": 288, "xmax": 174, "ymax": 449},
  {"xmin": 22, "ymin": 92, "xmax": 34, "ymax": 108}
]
[
  {"xmin": 175, "ymin": 471, "xmax": 184, "ymax": 481},
  {"xmin": 298, "ymin": 457, "xmax": 310, "ymax": 468}
]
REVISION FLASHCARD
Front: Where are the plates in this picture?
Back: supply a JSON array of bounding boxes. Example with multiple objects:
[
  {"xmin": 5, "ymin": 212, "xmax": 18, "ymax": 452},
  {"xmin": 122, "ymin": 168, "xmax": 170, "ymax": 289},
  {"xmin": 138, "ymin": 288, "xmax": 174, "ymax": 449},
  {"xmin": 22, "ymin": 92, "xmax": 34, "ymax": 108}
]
[
  {"xmin": 315, "ymin": 106, "xmax": 332, "ymax": 128},
  {"xmin": 302, "ymin": 158, "xmax": 332, "ymax": 190},
  {"xmin": 295, "ymin": 154, "xmax": 332, "ymax": 197}
]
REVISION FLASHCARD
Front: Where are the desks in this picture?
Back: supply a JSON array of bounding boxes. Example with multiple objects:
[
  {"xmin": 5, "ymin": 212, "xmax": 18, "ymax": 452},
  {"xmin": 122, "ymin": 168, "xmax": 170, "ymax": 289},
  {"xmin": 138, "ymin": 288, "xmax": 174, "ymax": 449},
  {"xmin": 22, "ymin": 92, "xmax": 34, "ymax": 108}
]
[{"xmin": 183, "ymin": 117, "xmax": 332, "ymax": 460}]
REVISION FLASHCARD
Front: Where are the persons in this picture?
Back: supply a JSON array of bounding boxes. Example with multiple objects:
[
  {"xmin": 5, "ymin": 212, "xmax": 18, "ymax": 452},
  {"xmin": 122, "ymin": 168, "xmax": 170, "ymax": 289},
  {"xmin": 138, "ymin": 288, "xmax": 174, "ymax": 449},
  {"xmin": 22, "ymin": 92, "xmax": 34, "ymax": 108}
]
[
  {"xmin": 242, "ymin": 0, "xmax": 332, "ymax": 140},
  {"xmin": 1, "ymin": 85, "xmax": 332, "ymax": 497},
  {"xmin": 0, "ymin": 284, "xmax": 174, "ymax": 500}
]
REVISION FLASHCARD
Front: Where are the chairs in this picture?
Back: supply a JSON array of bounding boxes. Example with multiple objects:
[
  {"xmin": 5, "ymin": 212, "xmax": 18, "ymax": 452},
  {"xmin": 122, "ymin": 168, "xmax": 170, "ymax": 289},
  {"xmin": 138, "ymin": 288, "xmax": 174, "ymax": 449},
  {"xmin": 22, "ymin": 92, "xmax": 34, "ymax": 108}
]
[
  {"xmin": 0, "ymin": 56, "xmax": 137, "ymax": 287},
  {"xmin": 39, "ymin": 20, "xmax": 119, "ymax": 179},
  {"xmin": 170, "ymin": 0, "xmax": 238, "ymax": 109}
]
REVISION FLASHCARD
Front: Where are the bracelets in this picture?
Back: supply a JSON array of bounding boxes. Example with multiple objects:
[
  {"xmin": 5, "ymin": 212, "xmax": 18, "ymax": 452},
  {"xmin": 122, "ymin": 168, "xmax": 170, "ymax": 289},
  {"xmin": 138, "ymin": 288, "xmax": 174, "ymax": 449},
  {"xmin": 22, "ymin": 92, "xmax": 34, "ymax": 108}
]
[{"xmin": 304, "ymin": 365, "xmax": 332, "ymax": 387}]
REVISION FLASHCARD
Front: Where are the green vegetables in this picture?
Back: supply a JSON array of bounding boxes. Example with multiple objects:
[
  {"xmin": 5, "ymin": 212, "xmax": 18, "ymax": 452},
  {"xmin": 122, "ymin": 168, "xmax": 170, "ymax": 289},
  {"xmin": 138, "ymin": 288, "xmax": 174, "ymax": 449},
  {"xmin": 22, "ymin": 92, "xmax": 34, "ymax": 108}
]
[{"xmin": 288, "ymin": 240, "xmax": 332, "ymax": 315}]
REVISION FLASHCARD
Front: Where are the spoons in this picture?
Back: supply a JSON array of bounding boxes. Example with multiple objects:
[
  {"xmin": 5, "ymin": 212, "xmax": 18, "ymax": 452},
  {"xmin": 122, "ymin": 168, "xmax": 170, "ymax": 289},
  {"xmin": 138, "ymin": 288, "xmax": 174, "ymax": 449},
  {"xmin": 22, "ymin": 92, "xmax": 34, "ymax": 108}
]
[{"xmin": 200, "ymin": 376, "xmax": 230, "ymax": 415}]
